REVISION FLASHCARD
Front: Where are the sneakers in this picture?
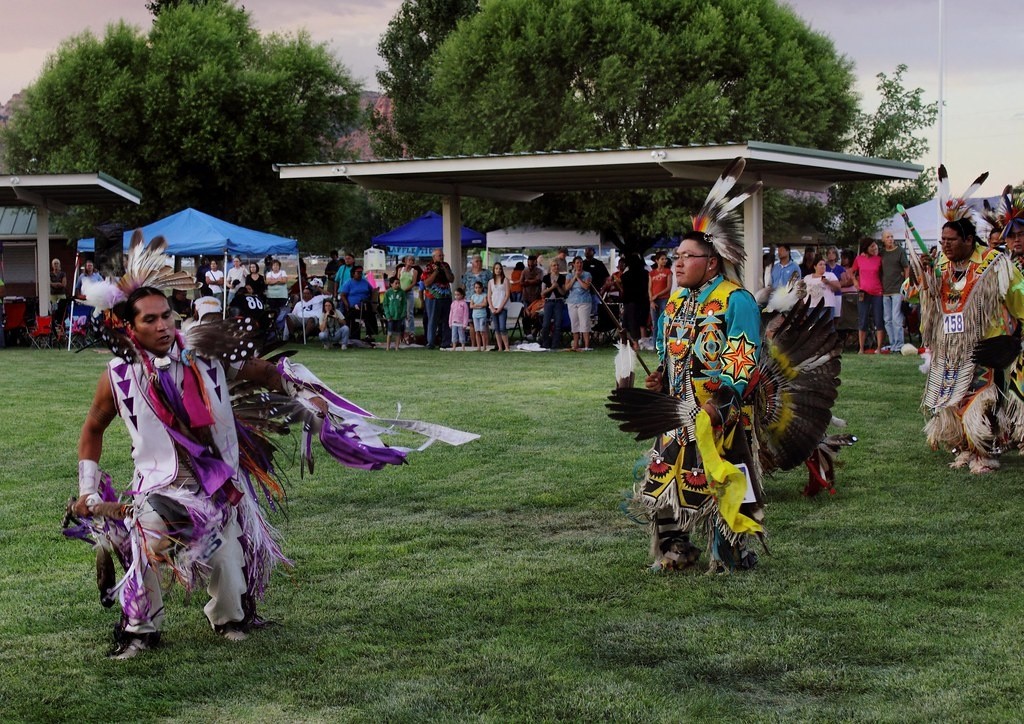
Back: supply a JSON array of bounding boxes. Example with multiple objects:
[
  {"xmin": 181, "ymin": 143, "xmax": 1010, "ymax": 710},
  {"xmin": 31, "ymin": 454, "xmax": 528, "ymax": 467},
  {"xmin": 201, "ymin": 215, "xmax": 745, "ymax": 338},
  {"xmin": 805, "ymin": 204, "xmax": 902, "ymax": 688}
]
[
  {"xmin": 323, "ymin": 342, "xmax": 331, "ymax": 351},
  {"xmin": 946, "ymin": 451, "xmax": 975, "ymax": 471},
  {"xmin": 341, "ymin": 343, "xmax": 347, "ymax": 352},
  {"xmin": 107, "ymin": 638, "xmax": 148, "ymax": 661},
  {"xmin": 222, "ymin": 626, "xmax": 249, "ymax": 643},
  {"xmin": 968, "ymin": 455, "xmax": 1001, "ymax": 475},
  {"xmin": 645, "ymin": 540, "xmax": 700, "ymax": 573}
]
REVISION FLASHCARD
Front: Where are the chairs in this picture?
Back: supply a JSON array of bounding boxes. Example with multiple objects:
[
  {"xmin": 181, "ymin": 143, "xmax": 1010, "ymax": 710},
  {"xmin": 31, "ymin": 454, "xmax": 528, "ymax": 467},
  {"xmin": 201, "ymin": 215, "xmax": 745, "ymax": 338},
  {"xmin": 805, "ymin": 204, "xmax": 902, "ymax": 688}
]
[{"xmin": 1, "ymin": 294, "xmax": 923, "ymax": 349}]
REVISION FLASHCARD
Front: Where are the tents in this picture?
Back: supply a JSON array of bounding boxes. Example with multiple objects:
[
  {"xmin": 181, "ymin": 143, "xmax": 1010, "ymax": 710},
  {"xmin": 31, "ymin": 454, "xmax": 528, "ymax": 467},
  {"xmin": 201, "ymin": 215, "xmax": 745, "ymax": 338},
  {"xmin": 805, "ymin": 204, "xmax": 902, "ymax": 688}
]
[
  {"xmin": 370, "ymin": 212, "xmax": 482, "ymax": 256},
  {"xmin": 484, "ymin": 226, "xmax": 602, "ymax": 272},
  {"xmin": 68, "ymin": 204, "xmax": 308, "ymax": 352},
  {"xmin": 862, "ymin": 196, "xmax": 998, "ymax": 256}
]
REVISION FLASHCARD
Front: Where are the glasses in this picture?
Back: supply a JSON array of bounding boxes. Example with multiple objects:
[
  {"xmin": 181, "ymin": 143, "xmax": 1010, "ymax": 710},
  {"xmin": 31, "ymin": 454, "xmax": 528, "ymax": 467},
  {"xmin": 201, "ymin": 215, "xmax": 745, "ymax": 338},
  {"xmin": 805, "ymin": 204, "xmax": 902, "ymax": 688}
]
[
  {"xmin": 671, "ymin": 253, "xmax": 712, "ymax": 260},
  {"xmin": 990, "ymin": 239, "xmax": 1009, "ymax": 248}
]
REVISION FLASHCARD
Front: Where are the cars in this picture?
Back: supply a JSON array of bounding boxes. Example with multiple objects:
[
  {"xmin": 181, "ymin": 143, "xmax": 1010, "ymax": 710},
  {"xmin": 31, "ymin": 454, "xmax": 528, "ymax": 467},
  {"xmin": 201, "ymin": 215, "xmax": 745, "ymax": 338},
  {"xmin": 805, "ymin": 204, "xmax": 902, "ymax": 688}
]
[
  {"xmin": 565, "ymin": 248, "xmax": 588, "ymax": 263},
  {"xmin": 497, "ymin": 253, "xmax": 530, "ymax": 268},
  {"xmin": 761, "ymin": 245, "xmax": 845, "ymax": 268}
]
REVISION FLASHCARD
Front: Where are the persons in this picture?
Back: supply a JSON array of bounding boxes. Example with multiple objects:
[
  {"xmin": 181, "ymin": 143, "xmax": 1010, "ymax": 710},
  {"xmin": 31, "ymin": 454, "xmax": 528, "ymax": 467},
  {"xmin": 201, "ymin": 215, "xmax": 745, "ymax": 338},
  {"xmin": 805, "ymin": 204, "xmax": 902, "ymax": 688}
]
[
  {"xmin": 71, "ymin": 290, "xmax": 265, "ymax": 662},
  {"xmin": 759, "ymin": 231, "xmax": 936, "ymax": 356},
  {"xmin": 617, "ymin": 233, "xmax": 764, "ymax": 575},
  {"xmin": 45, "ymin": 250, "xmax": 650, "ymax": 349},
  {"xmin": 646, "ymin": 252, "xmax": 672, "ymax": 355},
  {"xmin": 908, "ymin": 217, "xmax": 1024, "ymax": 474}
]
